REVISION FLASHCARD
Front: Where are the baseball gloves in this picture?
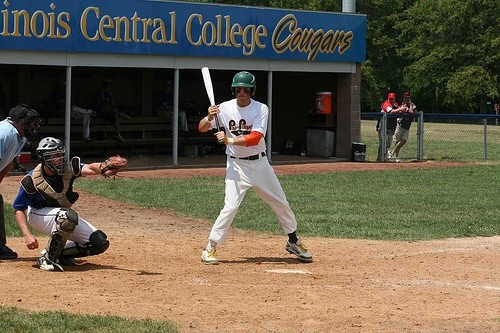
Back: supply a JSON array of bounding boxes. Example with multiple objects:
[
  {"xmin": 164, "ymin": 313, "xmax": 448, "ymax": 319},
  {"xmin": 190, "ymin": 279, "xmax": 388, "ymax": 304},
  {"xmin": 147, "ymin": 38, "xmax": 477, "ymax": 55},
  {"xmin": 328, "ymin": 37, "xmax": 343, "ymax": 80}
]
[{"xmin": 97, "ymin": 156, "xmax": 130, "ymax": 178}]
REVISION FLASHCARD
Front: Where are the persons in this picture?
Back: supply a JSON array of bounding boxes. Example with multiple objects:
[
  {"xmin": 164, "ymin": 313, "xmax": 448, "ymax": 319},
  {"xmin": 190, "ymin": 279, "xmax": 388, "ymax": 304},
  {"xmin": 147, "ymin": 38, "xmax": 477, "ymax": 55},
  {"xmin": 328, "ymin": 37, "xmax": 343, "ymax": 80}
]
[
  {"xmin": 96, "ymin": 78, "xmax": 131, "ymax": 141},
  {"xmin": 53, "ymin": 85, "xmax": 97, "ymax": 141},
  {"xmin": 0, "ymin": 106, "xmax": 42, "ymax": 258},
  {"xmin": 388, "ymin": 92, "xmax": 416, "ymax": 162},
  {"xmin": 13, "ymin": 137, "xmax": 128, "ymax": 270},
  {"xmin": 199, "ymin": 71, "xmax": 313, "ymax": 265},
  {"xmin": 156, "ymin": 79, "xmax": 174, "ymax": 119},
  {"xmin": 376, "ymin": 92, "xmax": 405, "ymax": 161}
]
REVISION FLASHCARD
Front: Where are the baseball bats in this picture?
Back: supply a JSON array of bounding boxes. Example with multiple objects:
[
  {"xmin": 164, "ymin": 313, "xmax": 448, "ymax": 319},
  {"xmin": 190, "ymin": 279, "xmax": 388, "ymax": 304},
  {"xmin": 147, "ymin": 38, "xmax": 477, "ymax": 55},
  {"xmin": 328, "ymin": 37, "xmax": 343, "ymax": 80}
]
[{"xmin": 201, "ymin": 65, "xmax": 225, "ymax": 145}]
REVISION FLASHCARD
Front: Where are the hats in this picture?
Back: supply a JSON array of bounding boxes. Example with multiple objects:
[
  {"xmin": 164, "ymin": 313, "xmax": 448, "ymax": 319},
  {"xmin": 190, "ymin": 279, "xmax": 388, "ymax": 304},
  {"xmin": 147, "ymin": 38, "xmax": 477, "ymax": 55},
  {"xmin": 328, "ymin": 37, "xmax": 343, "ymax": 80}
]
[{"xmin": 388, "ymin": 93, "xmax": 396, "ymax": 99}]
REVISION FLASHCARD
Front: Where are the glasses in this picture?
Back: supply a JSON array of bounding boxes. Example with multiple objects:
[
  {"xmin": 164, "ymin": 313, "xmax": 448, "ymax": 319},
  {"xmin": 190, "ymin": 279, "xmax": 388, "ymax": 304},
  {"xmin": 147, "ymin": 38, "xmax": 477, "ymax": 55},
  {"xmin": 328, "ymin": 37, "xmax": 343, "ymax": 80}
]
[
  {"xmin": 404, "ymin": 97, "xmax": 410, "ymax": 99},
  {"xmin": 235, "ymin": 88, "xmax": 250, "ymax": 95}
]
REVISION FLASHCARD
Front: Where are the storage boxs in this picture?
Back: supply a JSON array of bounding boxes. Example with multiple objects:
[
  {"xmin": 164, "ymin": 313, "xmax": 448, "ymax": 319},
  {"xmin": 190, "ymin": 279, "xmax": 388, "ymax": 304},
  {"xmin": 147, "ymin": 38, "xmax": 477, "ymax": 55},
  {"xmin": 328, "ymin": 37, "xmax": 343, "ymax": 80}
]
[
  {"xmin": 307, "ymin": 129, "xmax": 335, "ymax": 157},
  {"xmin": 20, "ymin": 152, "xmax": 32, "ymax": 163}
]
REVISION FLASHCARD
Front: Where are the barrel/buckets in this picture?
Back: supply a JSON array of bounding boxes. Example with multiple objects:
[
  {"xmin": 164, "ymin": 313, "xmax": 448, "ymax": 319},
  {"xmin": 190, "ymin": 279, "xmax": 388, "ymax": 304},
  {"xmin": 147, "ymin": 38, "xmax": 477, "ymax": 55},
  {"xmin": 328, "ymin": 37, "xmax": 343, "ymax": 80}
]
[{"xmin": 315, "ymin": 92, "xmax": 331, "ymax": 114}]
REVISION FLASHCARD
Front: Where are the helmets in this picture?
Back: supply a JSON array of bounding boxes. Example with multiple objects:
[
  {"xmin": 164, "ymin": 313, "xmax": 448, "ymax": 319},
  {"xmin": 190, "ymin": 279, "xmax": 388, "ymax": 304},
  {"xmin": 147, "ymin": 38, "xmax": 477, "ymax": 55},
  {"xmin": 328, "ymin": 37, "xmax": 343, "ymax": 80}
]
[
  {"xmin": 34, "ymin": 137, "xmax": 65, "ymax": 158},
  {"xmin": 8, "ymin": 103, "xmax": 41, "ymax": 126},
  {"xmin": 230, "ymin": 71, "xmax": 257, "ymax": 96}
]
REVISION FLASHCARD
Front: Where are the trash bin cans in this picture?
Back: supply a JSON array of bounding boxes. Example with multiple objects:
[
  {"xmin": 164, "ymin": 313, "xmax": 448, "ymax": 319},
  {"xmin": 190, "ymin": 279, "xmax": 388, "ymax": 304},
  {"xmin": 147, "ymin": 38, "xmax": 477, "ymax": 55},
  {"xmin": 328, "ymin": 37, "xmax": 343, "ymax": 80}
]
[{"xmin": 352, "ymin": 143, "xmax": 366, "ymax": 161}]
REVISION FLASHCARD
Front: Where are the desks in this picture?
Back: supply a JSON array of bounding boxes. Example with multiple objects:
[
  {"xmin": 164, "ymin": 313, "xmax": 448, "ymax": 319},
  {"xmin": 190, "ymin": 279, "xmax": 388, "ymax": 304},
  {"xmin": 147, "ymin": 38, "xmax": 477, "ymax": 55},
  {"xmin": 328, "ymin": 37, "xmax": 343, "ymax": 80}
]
[{"xmin": 302, "ymin": 124, "xmax": 338, "ymax": 156}]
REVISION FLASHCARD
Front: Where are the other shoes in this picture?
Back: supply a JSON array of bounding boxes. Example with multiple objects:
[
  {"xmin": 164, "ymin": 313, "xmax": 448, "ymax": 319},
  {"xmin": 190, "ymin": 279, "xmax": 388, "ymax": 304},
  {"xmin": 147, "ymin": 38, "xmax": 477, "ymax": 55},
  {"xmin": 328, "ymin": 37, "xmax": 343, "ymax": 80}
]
[
  {"xmin": 395, "ymin": 155, "xmax": 400, "ymax": 163},
  {"xmin": 0, "ymin": 245, "xmax": 19, "ymax": 259},
  {"xmin": 387, "ymin": 150, "xmax": 394, "ymax": 163},
  {"xmin": 61, "ymin": 255, "xmax": 75, "ymax": 266},
  {"xmin": 36, "ymin": 257, "xmax": 54, "ymax": 272}
]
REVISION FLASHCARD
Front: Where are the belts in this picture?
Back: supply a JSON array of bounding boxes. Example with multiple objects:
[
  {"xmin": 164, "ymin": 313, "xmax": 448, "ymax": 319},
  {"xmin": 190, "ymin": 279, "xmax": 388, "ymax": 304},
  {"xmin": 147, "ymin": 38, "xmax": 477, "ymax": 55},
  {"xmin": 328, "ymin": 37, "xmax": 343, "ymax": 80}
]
[{"xmin": 230, "ymin": 152, "xmax": 265, "ymax": 161}]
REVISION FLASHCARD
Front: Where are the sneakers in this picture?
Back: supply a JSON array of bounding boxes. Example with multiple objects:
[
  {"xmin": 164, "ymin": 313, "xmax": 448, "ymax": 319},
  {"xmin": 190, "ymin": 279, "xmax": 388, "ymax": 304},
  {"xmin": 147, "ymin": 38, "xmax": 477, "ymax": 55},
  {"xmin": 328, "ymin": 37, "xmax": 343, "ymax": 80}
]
[
  {"xmin": 200, "ymin": 248, "xmax": 219, "ymax": 265},
  {"xmin": 285, "ymin": 238, "xmax": 313, "ymax": 263}
]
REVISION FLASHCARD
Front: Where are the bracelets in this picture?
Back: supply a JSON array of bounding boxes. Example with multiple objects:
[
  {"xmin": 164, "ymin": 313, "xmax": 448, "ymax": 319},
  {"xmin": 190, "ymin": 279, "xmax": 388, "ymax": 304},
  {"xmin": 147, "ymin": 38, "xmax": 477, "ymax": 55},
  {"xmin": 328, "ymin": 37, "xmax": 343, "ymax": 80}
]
[
  {"xmin": 228, "ymin": 138, "xmax": 234, "ymax": 144},
  {"xmin": 205, "ymin": 116, "xmax": 211, "ymax": 123}
]
